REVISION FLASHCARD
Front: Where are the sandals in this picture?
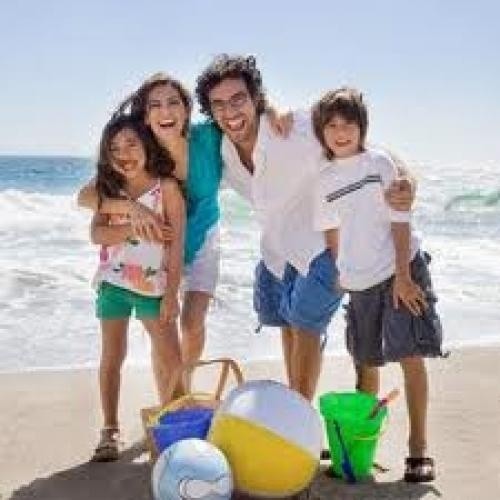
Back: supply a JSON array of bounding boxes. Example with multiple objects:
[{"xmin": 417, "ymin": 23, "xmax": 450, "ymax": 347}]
[
  {"xmin": 94, "ymin": 426, "xmax": 122, "ymax": 462},
  {"xmin": 402, "ymin": 457, "xmax": 439, "ymax": 484}
]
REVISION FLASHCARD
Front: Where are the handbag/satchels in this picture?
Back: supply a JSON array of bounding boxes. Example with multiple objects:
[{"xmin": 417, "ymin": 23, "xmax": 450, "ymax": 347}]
[{"xmin": 140, "ymin": 353, "xmax": 248, "ymax": 458}]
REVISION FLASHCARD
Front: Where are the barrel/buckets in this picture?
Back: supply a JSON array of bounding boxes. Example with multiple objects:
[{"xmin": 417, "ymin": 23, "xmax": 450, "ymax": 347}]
[
  {"xmin": 146, "ymin": 393, "xmax": 212, "ymax": 454},
  {"xmin": 319, "ymin": 390, "xmax": 389, "ymax": 481}
]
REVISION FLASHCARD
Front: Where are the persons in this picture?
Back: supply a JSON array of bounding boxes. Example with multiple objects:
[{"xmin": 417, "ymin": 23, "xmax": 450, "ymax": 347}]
[
  {"xmin": 73, "ymin": 70, "xmax": 296, "ymax": 451},
  {"xmin": 196, "ymin": 51, "xmax": 418, "ymax": 403},
  {"xmin": 90, "ymin": 114, "xmax": 188, "ymax": 461},
  {"xmin": 308, "ymin": 85, "xmax": 452, "ymax": 483}
]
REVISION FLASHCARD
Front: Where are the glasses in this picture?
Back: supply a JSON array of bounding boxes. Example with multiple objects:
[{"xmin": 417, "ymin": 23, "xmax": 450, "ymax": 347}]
[{"xmin": 206, "ymin": 92, "xmax": 254, "ymax": 108}]
